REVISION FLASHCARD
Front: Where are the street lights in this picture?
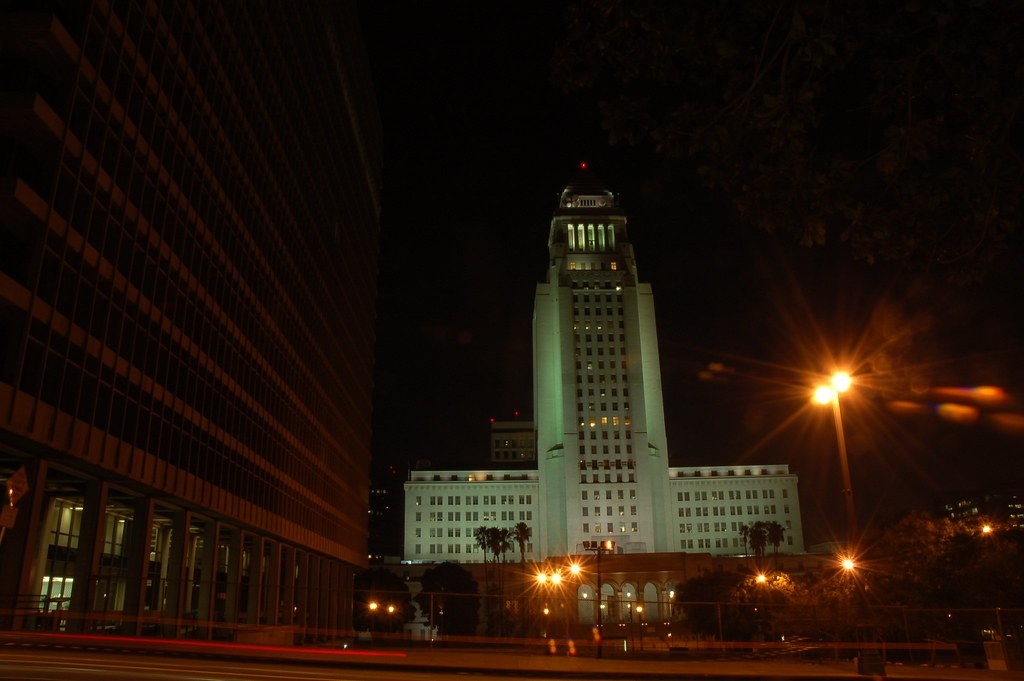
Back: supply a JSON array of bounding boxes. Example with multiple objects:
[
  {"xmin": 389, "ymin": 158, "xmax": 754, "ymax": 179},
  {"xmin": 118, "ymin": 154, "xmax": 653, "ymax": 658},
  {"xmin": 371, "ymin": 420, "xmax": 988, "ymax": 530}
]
[
  {"xmin": 582, "ymin": 540, "xmax": 616, "ymax": 658},
  {"xmin": 809, "ymin": 366, "xmax": 887, "ymax": 676}
]
